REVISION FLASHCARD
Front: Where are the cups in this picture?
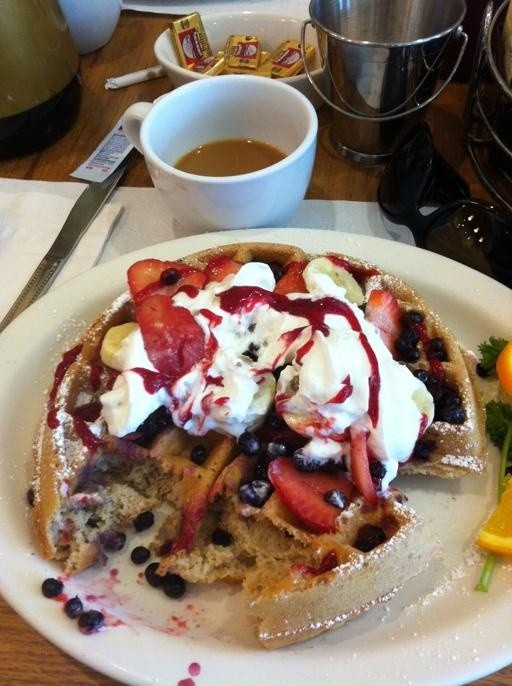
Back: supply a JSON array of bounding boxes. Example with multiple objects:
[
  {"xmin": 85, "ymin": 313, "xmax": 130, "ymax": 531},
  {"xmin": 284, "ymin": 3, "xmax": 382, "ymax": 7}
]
[{"xmin": 122, "ymin": 76, "xmax": 319, "ymax": 230}]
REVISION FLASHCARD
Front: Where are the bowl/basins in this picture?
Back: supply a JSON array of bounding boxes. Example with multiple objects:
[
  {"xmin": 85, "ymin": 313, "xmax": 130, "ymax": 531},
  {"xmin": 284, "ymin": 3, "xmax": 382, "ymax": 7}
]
[{"xmin": 149, "ymin": 12, "xmax": 335, "ymax": 89}]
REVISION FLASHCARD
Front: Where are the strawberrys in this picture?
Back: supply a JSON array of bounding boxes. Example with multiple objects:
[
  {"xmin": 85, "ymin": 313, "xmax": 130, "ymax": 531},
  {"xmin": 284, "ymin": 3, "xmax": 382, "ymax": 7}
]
[
  {"xmin": 134, "ymin": 293, "xmax": 204, "ymax": 378},
  {"xmin": 347, "ymin": 427, "xmax": 381, "ymax": 510},
  {"xmin": 364, "ymin": 288, "xmax": 405, "ymax": 358},
  {"xmin": 265, "ymin": 453, "xmax": 354, "ymax": 533},
  {"xmin": 126, "ymin": 254, "xmax": 354, "ymax": 303}
]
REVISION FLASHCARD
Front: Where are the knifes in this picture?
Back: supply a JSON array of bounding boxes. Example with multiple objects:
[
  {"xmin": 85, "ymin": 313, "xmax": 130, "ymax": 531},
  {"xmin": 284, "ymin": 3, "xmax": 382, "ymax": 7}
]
[{"xmin": 1, "ymin": 163, "xmax": 141, "ymax": 334}]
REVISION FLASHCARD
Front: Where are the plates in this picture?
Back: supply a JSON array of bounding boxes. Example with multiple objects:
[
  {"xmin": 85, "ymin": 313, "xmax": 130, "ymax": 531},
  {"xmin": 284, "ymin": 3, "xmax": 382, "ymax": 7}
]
[{"xmin": 0, "ymin": 229, "xmax": 512, "ymax": 686}]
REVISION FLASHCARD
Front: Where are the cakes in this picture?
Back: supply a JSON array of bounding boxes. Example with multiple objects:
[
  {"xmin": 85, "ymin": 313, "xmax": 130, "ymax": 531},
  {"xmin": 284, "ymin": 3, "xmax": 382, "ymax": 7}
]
[{"xmin": 29, "ymin": 244, "xmax": 489, "ymax": 652}]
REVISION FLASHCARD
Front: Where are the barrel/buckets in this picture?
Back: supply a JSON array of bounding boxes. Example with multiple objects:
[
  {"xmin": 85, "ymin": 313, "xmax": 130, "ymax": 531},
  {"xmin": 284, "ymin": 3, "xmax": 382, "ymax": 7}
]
[
  {"xmin": 300, "ymin": 0, "xmax": 470, "ymax": 166},
  {"xmin": 464, "ymin": 1, "xmax": 512, "ymax": 218}
]
